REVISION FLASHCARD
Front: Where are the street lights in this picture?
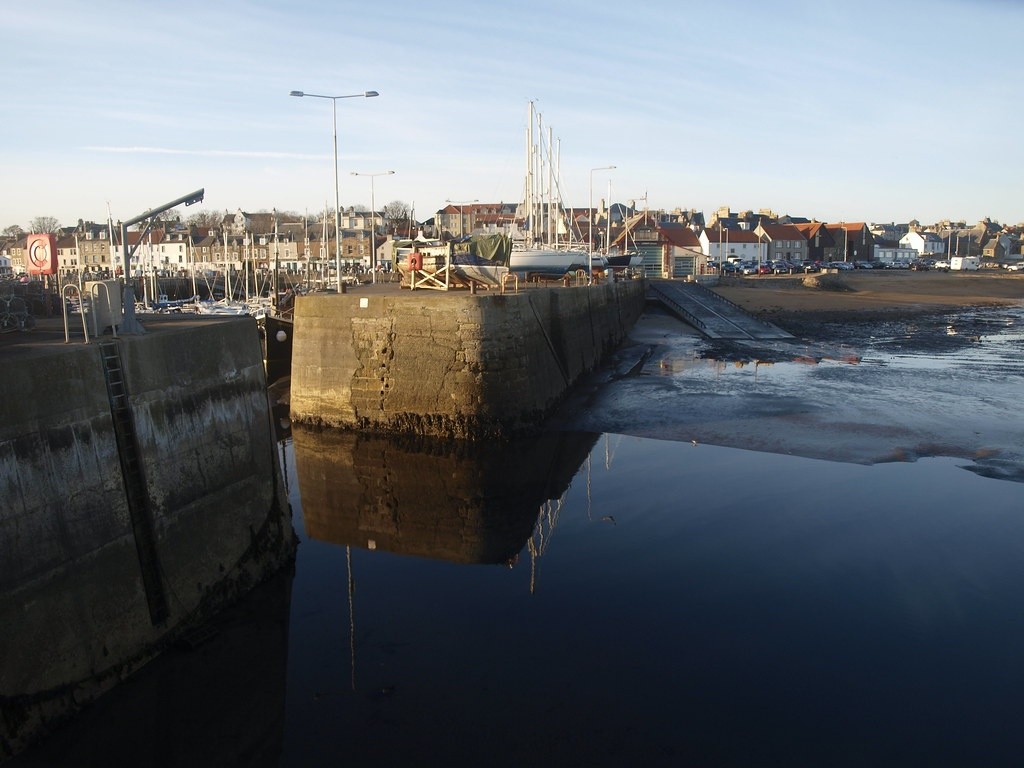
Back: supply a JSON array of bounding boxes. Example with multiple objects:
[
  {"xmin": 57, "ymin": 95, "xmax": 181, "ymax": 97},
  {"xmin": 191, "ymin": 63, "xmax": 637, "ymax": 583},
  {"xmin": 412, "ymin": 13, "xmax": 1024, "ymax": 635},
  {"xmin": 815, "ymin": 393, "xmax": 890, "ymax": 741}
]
[
  {"xmin": 289, "ymin": 89, "xmax": 381, "ymax": 294},
  {"xmin": 841, "ymin": 226, "xmax": 848, "ymax": 263},
  {"xmin": 587, "ymin": 165, "xmax": 618, "ymax": 285},
  {"xmin": 723, "ymin": 228, "xmax": 729, "ymax": 261},
  {"xmin": 350, "ymin": 170, "xmax": 396, "ymax": 284},
  {"xmin": 442, "ymin": 199, "xmax": 482, "ymax": 234}
]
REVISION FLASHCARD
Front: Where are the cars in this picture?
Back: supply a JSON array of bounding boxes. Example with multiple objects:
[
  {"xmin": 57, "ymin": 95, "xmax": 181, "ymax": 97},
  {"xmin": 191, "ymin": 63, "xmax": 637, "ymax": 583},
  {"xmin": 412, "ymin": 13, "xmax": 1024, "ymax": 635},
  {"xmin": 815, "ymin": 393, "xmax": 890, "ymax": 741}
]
[
  {"xmin": 1007, "ymin": 262, "xmax": 1024, "ymax": 272},
  {"xmin": 705, "ymin": 254, "xmax": 952, "ymax": 273}
]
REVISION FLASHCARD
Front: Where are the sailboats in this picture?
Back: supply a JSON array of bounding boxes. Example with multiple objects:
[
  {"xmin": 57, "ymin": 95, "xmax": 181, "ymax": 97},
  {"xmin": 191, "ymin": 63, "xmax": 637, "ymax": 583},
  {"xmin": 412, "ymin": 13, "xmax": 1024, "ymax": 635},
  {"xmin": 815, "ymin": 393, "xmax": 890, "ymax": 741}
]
[{"xmin": 124, "ymin": 98, "xmax": 648, "ymax": 319}]
